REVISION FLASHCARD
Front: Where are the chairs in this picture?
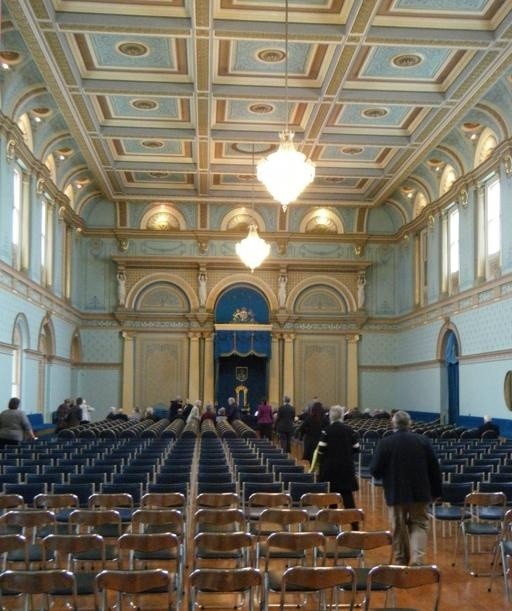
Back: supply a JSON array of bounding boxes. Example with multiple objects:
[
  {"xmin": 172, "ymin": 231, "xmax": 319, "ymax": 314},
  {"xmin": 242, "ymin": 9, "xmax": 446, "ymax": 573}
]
[{"xmin": 0, "ymin": 407, "xmax": 512, "ymax": 610}]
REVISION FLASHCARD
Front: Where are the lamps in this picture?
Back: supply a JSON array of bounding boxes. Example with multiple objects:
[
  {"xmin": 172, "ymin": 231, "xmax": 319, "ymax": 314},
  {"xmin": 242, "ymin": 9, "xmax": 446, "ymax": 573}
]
[
  {"xmin": 254, "ymin": 0, "xmax": 317, "ymax": 216},
  {"xmin": 232, "ymin": 203, "xmax": 275, "ymax": 277}
]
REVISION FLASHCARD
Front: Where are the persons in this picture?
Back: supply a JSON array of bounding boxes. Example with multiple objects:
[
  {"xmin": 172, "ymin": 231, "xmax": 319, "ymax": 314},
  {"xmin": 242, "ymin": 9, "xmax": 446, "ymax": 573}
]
[
  {"xmin": 139, "ymin": 394, "xmax": 239, "ymax": 424},
  {"xmin": 368, "ymin": 411, "xmax": 442, "ymax": 566},
  {"xmin": 343, "ymin": 405, "xmax": 399, "ymax": 419},
  {"xmin": 480, "ymin": 413, "xmax": 500, "ymax": 436},
  {"xmin": 255, "ymin": 395, "xmax": 329, "ymax": 463},
  {"xmin": 317, "ymin": 402, "xmax": 362, "ymax": 534},
  {"xmin": 0, "ymin": 396, "xmax": 37, "ymax": 449},
  {"xmin": 55, "ymin": 396, "xmax": 95, "ymax": 430},
  {"xmin": 107, "ymin": 407, "xmax": 129, "ymax": 421}
]
